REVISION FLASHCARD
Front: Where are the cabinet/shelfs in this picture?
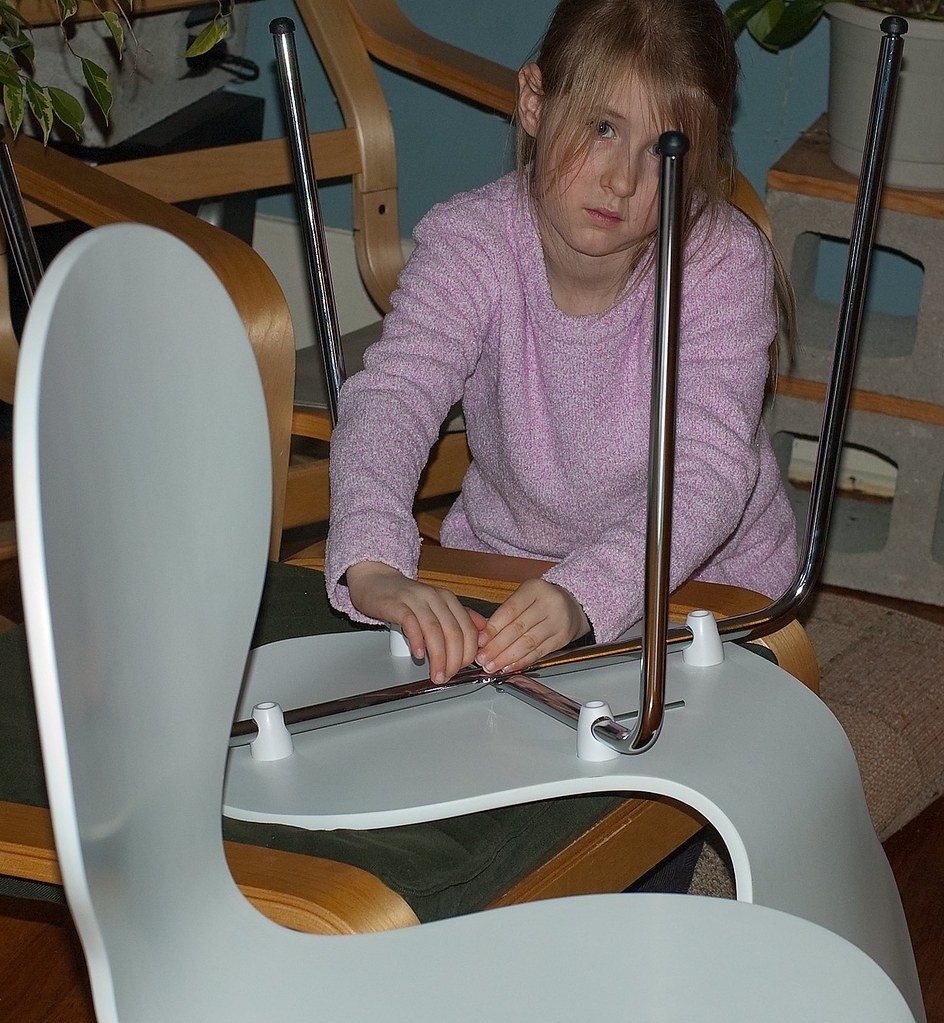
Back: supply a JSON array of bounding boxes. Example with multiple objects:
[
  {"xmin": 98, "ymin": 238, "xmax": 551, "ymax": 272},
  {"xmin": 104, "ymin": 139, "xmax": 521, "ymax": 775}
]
[
  {"xmin": 764, "ymin": 112, "xmax": 944, "ymax": 608},
  {"xmin": 0, "ymin": 91, "xmax": 265, "ymax": 340}
]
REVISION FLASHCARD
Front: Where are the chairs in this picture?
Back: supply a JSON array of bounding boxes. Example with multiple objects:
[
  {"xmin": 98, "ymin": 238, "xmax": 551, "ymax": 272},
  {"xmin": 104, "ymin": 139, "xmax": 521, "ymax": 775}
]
[{"xmin": 0, "ymin": 0, "xmax": 944, "ymax": 1023}]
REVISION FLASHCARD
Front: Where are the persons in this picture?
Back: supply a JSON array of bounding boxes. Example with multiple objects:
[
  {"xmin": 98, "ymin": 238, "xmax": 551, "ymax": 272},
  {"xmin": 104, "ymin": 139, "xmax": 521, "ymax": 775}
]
[{"xmin": 323, "ymin": 1, "xmax": 800, "ymax": 896}]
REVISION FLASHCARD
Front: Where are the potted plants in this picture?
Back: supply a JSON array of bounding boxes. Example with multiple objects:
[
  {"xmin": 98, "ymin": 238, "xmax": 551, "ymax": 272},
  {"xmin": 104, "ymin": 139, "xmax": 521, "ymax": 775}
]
[{"xmin": 723, "ymin": 0, "xmax": 944, "ymax": 189}]
